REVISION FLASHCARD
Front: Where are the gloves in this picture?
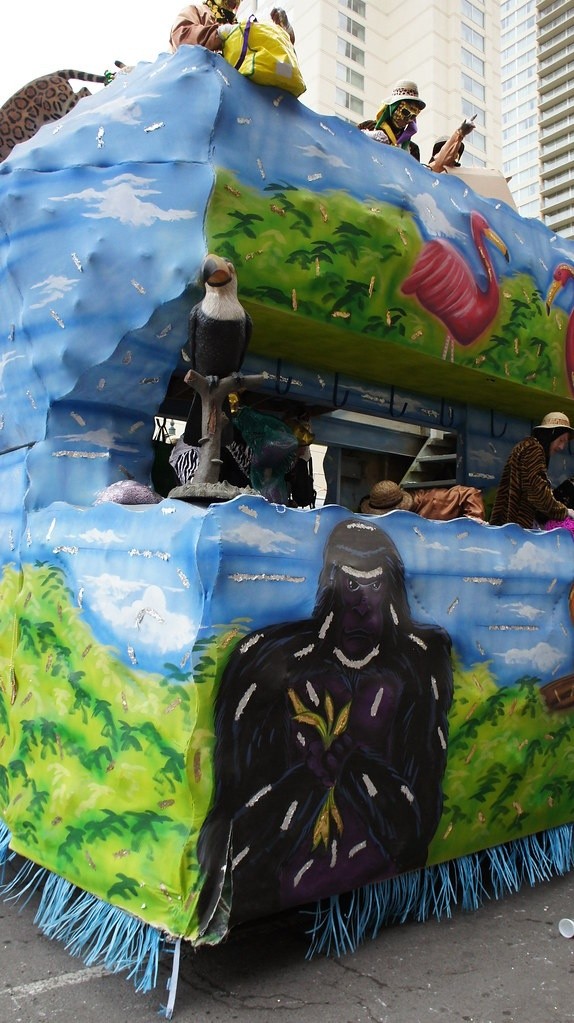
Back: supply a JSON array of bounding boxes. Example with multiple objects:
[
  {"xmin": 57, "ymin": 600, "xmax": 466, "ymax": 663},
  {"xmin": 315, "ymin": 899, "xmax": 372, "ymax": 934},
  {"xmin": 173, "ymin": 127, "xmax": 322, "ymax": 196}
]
[{"xmin": 217, "ymin": 23, "xmax": 239, "ymax": 40}]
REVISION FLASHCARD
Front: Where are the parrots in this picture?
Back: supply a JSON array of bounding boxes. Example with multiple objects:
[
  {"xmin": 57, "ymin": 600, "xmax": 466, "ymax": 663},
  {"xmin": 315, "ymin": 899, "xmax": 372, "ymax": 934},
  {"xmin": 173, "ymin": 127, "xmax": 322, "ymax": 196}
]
[{"xmin": 180, "ymin": 253, "xmax": 253, "ymax": 447}]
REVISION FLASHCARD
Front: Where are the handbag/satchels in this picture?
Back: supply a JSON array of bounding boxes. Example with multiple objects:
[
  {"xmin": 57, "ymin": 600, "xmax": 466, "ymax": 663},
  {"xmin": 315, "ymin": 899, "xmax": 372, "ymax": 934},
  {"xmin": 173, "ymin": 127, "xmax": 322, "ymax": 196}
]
[{"xmin": 219, "ymin": 13, "xmax": 307, "ymax": 98}]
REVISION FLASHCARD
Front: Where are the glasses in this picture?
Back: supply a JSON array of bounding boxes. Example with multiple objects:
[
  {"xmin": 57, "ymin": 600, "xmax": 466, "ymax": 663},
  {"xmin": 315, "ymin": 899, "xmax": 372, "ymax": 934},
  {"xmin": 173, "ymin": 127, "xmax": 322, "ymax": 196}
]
[{"xmin": 401, "ymin": 109, "xmax": 417, "ymax": 120}]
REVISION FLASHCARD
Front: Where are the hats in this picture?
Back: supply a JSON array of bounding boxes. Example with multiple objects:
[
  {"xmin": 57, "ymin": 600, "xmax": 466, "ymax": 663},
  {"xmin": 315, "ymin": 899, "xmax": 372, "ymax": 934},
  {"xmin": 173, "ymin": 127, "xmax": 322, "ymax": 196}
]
[
  {"xmin": 382, "ymin": 80, "xmax": 426, "ymax": 110},
  {"xmin": 432, "ymin": 135, "xmax": 465, "ymax": 161},
  {"xmin": 532, "ymin": 412, "xmax": 574, "ymax": 440},
  {"xmin": 359, "ymin": 480, "xmax": 413, "ymax": 515}
]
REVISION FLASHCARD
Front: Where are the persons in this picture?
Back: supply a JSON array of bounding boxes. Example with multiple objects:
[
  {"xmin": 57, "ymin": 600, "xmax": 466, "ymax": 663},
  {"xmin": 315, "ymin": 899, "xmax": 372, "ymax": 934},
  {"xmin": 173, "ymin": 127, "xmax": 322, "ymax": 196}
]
[
  {"xmin": 351, "ymin": 479, "xmax": 487, "ymax": 525},
  {"xmin": 489, "ymin": 412, "xmax": 574, "ymax": 529},
  {"xmin": 423, "ymin": 113, "xmax": 478, "ymax": 173},
  {"xmin": 358, "ymin": 80, "xmax": 426, "ymax": 162},
  {"xmin": 169, "ymin": 0, "xmax": 295, "ymax": 55}
]
